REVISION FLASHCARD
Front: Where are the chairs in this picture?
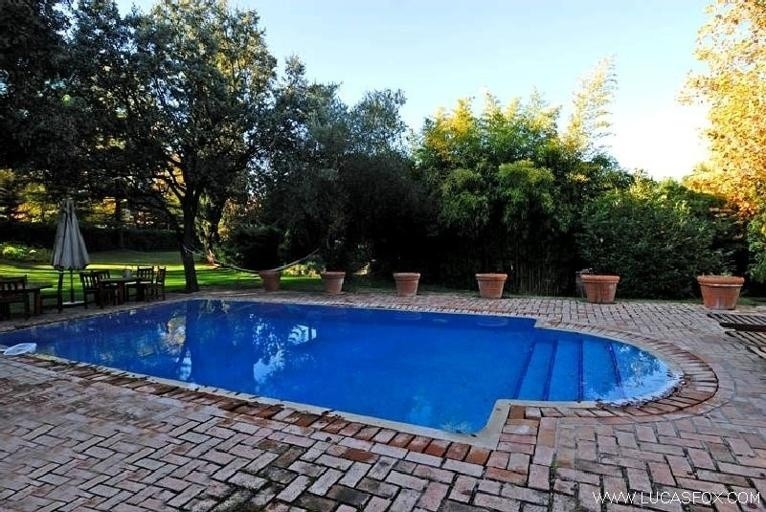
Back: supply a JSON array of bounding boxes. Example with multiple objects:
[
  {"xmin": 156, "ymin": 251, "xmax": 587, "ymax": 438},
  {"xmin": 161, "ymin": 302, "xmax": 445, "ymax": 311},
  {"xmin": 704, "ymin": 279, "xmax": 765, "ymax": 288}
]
[{"xmin": 0, "ymin": 264, "xmax": 166, "ymax": 321}]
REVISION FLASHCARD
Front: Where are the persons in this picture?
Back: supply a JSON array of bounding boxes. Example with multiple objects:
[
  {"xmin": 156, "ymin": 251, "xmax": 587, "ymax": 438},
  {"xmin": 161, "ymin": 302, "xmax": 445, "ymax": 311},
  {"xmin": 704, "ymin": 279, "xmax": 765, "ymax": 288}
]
[{"xmin": 207, "ymin": 249, "xmax": 215, "ymax": 265}]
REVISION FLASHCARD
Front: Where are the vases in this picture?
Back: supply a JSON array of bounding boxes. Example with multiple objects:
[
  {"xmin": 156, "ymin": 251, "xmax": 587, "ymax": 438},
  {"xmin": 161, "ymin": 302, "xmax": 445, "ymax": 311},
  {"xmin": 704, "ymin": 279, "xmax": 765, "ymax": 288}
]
[
  {"xmin": 258, "ymin": 270, "xmax": 281, "ymax": 292},
  {"xmin": 697, "ymin": 273, "xmax": 746, "ymax": 310},
  {"xmin": 576, "ymin": 273, "xmax": 621, "ymax": 304},
  {"xmin": 320, "ymin": 271, "xmax": 346, "ymax": 294},
  {"xmin": 475, "ymin": 273, "xmax": 508, "ymax": 300},
  {"xmin": 392, "ymin": 272, "xmax": 421, "ymax": 297}
]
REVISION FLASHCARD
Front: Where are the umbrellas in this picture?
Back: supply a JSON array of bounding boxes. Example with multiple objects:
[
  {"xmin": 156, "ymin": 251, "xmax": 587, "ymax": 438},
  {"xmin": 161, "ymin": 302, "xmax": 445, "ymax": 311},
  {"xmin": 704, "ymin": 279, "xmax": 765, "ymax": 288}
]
[{"xmin": 51, "ymin": 198, "xmax": 90, "ymax": 302}]
